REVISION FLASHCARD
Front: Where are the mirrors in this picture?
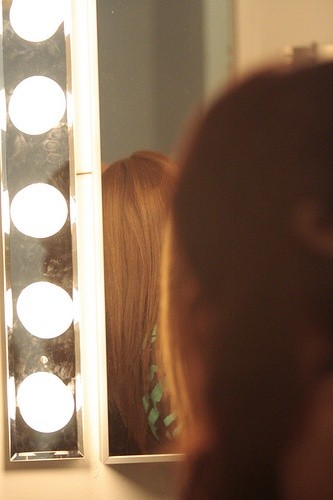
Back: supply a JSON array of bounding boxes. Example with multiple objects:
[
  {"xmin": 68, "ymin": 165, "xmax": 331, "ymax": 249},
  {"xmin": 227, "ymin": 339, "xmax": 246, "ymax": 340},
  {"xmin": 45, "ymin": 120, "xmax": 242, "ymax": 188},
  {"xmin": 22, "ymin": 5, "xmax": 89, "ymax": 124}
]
[{"xmin": 76, "ymin": 0, "xmax": 333, "ymax": 465}]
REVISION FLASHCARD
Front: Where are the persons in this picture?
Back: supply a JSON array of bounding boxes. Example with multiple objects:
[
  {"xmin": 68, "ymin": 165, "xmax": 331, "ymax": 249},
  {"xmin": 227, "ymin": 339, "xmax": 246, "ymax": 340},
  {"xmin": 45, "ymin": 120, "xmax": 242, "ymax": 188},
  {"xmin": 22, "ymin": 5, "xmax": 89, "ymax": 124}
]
[
  {"xmin": 99, "ymin": 153, "xmax": 193, "ymax": 454},
  {"xmin": 160, "ymin": 52, "xmax": 333, "ymax": 499},
  {"xmin": 40, "ymin": 158, "xmax": 109, "ymax": 452}
]
